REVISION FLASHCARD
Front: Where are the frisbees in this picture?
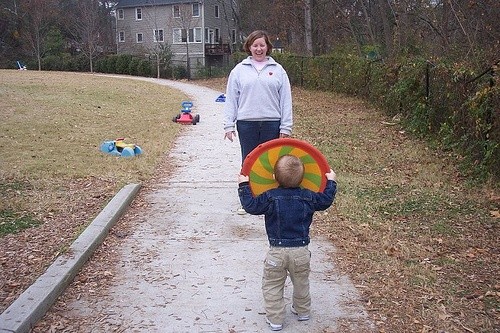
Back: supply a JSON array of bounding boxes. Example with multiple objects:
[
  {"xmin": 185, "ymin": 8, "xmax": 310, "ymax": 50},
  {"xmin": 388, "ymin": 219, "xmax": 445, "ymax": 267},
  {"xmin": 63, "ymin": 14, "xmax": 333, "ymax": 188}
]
[{"xmin": 240, "ymin": 137, "xmax": 329, "ymax": 198}]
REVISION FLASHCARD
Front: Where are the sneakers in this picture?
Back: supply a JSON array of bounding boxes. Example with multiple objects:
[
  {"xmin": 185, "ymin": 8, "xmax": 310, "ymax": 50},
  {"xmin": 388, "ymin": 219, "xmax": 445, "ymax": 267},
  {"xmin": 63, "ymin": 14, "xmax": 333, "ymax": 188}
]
[
  {"xmin": 237, "ymin": 203, "xmax": 249, "ymax": 214},
  {"xmin": 291, "ymin": 303, "xmax": 310, "ymax": 321},
  {"xmin": 265, "ymin": 317, "xmax": 282, "ymax": 331}
]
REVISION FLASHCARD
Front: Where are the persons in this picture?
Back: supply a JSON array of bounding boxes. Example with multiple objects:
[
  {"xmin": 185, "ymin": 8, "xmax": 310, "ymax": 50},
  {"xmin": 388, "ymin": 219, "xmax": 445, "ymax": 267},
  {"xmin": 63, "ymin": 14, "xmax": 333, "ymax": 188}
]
[
  {"xmin": 222, "ymin": 28, "xmax": 294, "ymax": 215},
  {"xmin": 235, "ymin": 153, "xmax": 339, "ymax": 332}
]
